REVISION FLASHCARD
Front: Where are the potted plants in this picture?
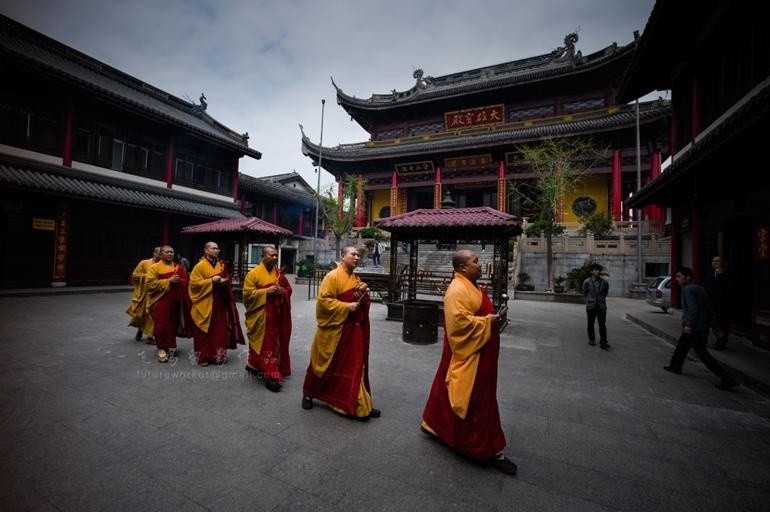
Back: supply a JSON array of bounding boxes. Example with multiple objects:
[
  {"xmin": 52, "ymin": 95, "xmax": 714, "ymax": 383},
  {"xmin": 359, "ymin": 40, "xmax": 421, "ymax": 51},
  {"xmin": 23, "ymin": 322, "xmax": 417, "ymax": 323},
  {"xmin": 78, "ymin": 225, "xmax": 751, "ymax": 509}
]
[
  {"xmin": 553, "ymin": 275, "xmax": 567, "ymax": 292},
  {"xmin": 295, "ymin": 261, "xmax": 305, "ymax": 277},
  {"xmin": 516, "ymin": 272, "xmax": 535, "ymax": 291},
  {"xmin": 305, "ymin": 262, "xmax": 317, "ymax": 279}
]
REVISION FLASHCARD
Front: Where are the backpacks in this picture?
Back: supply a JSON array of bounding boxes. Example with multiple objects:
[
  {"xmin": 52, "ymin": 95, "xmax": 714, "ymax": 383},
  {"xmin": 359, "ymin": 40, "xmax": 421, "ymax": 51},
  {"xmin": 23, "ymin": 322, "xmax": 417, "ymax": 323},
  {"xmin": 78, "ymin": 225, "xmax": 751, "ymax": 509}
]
[{"xmin": 693, "ymin": 285, "xmax": 714, "ymax": 320}]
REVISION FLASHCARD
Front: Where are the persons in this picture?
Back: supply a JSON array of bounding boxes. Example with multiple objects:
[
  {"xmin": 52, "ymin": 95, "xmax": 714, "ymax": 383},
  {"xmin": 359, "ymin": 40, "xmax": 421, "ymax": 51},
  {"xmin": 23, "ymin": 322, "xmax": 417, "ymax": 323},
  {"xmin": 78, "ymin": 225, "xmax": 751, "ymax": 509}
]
[
  {"xmin": 477, "ymin": 239, "xmax": 487, "ymax": 251},
  {"xmin": 301, "ymin": 245, "xmax": 382, "ymax": 422},
  {"xmin": 242, "ymin": 244, "xmax": 292, "ymax": 392},
  {"xmin": 177, "ymin": 249, "xmax": 190, "ymax": 273},
  {"xmin": 582, "ymin": 262, "xmax": 612, "ymax": 352},
  {"xmin": 399, "ymin": 240, "xmax": 409, "ymax": 256},
  {"xmin": 371, "ymin": 238, "xmax": 382, "ymax": 268},
  {"xmin": 125, "ymin": 245, "xmax": 162, "ymax": 345},
  {"xmin": 662, "ymin": 266, "xmax": 740, "ymax": 391},
  {"xmin": 187, "ymin": 240, "xmax": 247, "ymax": 367},
  {"xmin": 420, "ymin": 248, "xmax": 518, "ymax": 478},
  {"xmin": 143, "ymin": 243, "xmax": 194, "ymax": 363},
  {"xmin": 697, "ymin": 255, "xmax": 732, "ymax": 351}
]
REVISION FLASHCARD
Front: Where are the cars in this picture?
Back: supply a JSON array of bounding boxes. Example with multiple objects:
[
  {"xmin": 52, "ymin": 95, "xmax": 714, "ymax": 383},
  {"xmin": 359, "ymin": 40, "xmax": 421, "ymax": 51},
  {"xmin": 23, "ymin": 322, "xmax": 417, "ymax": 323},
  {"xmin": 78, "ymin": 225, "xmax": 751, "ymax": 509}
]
[{"xmin": 647, "ymin": 274, "xmax": 671, "ymax": 312}]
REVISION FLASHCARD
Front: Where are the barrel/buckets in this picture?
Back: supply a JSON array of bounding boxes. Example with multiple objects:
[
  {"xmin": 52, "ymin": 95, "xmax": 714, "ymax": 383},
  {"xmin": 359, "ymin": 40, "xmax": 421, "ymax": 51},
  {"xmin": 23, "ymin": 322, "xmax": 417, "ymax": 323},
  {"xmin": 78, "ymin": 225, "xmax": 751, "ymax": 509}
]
[{"xmin": 402, "ymin": 304, "xmax": 438, "ymax": 344}]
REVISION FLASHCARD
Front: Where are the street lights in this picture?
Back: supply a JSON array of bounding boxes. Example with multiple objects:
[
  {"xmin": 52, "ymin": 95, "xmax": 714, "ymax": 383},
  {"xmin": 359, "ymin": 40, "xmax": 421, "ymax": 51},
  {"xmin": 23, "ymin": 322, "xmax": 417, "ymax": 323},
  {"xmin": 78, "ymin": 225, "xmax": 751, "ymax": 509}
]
[{"xmin": 311, "ymin": 98, "xmax": 328, "ymax": 264}]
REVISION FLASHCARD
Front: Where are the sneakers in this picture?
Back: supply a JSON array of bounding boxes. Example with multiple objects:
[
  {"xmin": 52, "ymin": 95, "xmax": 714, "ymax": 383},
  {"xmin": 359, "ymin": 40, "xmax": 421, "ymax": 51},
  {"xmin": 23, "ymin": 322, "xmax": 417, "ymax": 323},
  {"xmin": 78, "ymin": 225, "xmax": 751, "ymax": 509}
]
[
  {"xmin": 589, "ymin": 342, "xmax": 595, "ymax": 346},
  {"xmin": 245, "ymin": 365, "xmax": 260, "ymax": 377},
  {"xmin": 714, "ymin": 376, "xmax": 739, "ymax": 391},
  {"xmin": 264, "ymin": 378, "xmax": 282, "ymax": 392},
  {"xmin": 146, "ymin": 337, "xmax": 157, "ymax": 345},
  {"xmin": 491, "ymin": 455, "xmax": 518, "ymax": 475},
  {"xmin": 420, "ymin": 426, "xmax": 441, "ymax": 443},
  {"xmin": 199, "ymin": 361, "xmax": 209, "ymax": 367},
  {"xmin": 136, "ymin": 330, "xmax": 142, "ymax": 342},
  {"xmin": 663, "ymin": 366, "xmax": 681, "ymax": 374},
  {"xmin": 302, "ymin": 396, "xmax": 313, "ymax": 410},
  {"xmin": 601, "ymin": 344, "xmax": 611, "ymax": 350},
  {"xmin": 156, "ymin": 349, "xmax": 170, "ymax": 363},
  {"xmin": 369, "ymin": 407, "xmax": 381, "ymax": 418}
]
